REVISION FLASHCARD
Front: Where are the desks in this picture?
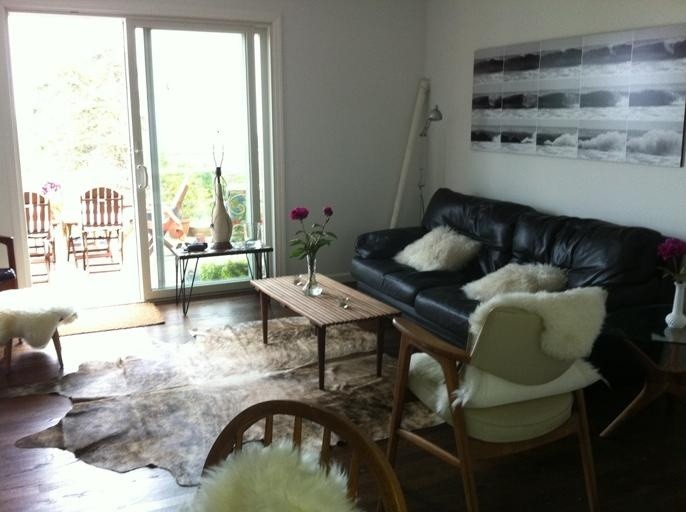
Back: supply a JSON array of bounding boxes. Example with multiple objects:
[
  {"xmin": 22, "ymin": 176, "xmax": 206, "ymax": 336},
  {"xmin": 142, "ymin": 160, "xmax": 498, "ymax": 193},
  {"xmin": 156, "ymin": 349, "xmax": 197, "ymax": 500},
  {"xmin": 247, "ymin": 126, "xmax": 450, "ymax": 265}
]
[
  {"xmin": 249, "ymin": 273, "xmax": 402, "ymax": 389},
  {"xmin": 169, "ymin": 239, "xmax": 273, "ymax": 318}
]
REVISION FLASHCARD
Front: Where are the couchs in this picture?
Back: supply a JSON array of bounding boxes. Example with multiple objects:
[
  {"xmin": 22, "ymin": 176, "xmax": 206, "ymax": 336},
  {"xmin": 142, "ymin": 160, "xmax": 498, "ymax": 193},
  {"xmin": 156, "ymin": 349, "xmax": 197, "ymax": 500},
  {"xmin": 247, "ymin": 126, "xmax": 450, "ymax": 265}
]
[{"xmin": 349, "ymin": 187, "xmax": 670, "ymax": 423}]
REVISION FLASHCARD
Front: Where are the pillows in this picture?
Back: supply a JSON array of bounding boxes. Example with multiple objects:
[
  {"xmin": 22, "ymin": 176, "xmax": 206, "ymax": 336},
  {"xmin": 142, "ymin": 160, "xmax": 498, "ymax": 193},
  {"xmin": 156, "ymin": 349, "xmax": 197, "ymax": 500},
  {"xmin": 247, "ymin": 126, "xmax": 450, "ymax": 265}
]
[{"xmin": 396, "ymin": 224, "xmax": 568, "ymax": 302}]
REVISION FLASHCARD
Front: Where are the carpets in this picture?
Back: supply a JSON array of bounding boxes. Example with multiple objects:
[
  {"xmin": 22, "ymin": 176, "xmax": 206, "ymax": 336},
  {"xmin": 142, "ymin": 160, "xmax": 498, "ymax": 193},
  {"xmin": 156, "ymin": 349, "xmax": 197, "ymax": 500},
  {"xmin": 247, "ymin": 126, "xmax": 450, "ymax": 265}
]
[
  {"xmin": 56, "ymin": 300, "xmax": 166, "ymax": 337},
  {"xmin": 0, "ymin": 315, "xmax": 448, "ymax": 486}
]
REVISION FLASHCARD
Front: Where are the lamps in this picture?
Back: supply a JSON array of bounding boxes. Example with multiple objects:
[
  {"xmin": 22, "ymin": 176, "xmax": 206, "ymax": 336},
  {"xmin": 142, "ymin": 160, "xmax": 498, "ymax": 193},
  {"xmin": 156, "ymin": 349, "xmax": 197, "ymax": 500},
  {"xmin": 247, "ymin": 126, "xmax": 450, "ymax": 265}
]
[{"xmin": 418, "ymin": 101, "xmax": 443, "ymax": 226}]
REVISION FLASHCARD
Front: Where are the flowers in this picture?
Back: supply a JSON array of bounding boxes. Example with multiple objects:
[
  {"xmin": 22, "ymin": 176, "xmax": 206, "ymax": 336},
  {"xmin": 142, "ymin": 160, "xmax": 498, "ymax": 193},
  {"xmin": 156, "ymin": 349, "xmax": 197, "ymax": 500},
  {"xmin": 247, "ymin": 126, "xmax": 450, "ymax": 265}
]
[
  {"xmin": 288, "ymin": 204, "xmax": 337, "ymax": 256},
  {"xmin": 653, "ymin": 236, "xmax": 685, "ymax": 285}
]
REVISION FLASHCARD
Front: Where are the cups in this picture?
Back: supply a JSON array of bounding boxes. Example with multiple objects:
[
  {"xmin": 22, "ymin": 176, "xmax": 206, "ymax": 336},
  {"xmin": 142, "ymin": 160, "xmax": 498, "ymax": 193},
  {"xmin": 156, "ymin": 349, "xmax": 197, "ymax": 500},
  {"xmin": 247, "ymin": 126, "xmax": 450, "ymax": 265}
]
[
  {"xmin": 336, "ymin": 297, "xmax": 348, "ymax": 309},
  {"xmin": 291, "ymin": 273, "xmax": 303, "ymax": 285}
]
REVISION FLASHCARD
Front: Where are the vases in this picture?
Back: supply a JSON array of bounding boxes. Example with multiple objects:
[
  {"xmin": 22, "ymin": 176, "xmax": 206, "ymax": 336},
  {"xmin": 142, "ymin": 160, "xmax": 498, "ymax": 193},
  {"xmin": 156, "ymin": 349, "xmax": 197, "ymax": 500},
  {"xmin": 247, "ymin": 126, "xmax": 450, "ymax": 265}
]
[
  {"xmin": 210, "ymin": 183, "xmax": 235, "ymax": 244},
  {"xmin": 303, "ymin": 255, "xmax": 328, "ymax": 298},
  {"xmin": 664, "ymin": 284, "xmax": 686, "ymax": 329}
]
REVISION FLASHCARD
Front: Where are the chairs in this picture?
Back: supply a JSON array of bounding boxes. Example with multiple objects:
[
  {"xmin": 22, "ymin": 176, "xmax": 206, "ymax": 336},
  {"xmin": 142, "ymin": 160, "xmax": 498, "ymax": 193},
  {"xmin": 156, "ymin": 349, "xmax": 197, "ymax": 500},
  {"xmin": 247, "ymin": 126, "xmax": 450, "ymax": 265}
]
[
  {"xmin": 0, "ymin": 235, "xmax": 79, "ymax": 382},
  {"xmin": 21, "ymin": 187, "xmax": 124, "ymax": 282},
  {"xmin": 192, "ymin": 400, "xmax": 409, "ymax": 512},
  {"xmin": 383, "ymin": 285, "xmax": 609, "ymax": 512}
]
